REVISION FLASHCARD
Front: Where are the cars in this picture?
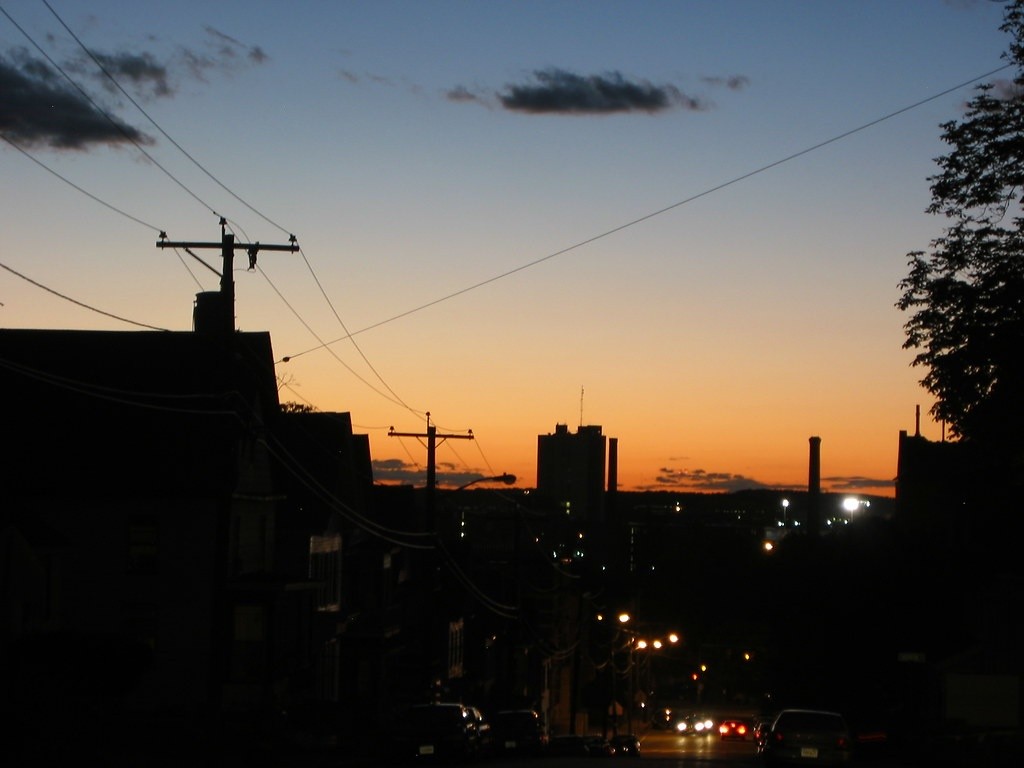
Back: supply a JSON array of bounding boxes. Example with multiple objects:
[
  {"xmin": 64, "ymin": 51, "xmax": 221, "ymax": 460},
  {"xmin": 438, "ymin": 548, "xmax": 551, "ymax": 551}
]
[
  {"xmin": 651, "ymin": 706, "xmax": 776, "ymax": 746},
  {"xmin": 275, "ymin": 702, "xmax": 646, "ymax": 764},
  {"xmin": 762, "ymin": 708, "xmax": 852, "ymax": 766}
]
